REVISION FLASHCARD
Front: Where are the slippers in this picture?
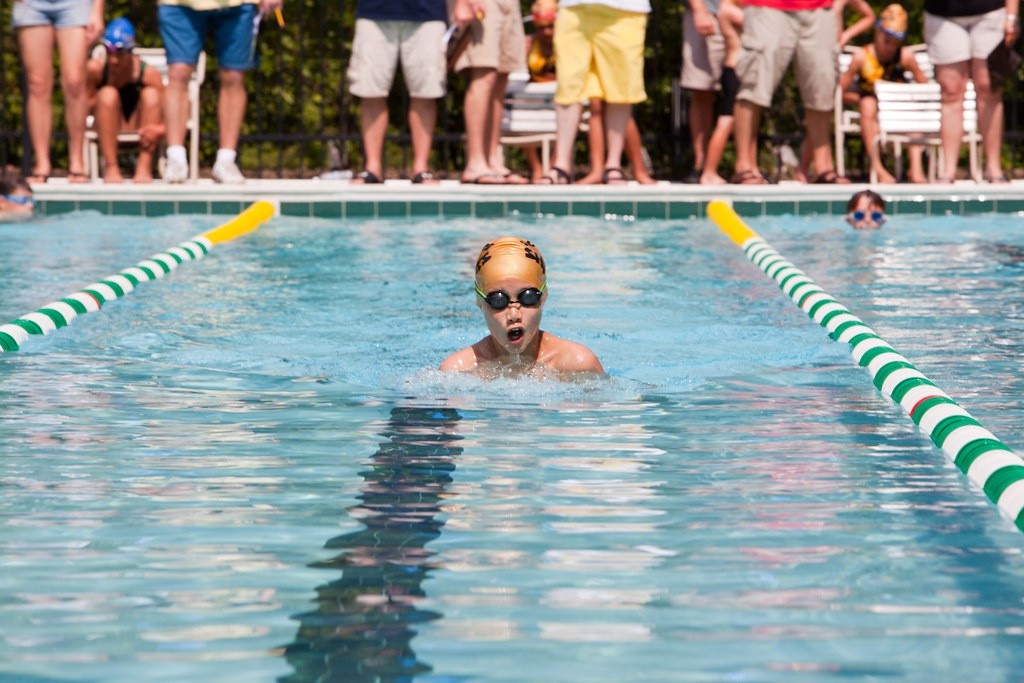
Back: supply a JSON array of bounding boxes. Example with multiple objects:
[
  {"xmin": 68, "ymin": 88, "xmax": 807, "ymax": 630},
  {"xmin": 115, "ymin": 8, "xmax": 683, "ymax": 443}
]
[
  {"xmin": 541, "ymin": 167, "xmax": 571, "ymax": 184},
  {"xmin": 733, "ymin": 169, "xmax": 768, "ymax": 184},
  {"xmin": 353, "ymin": 171, "xmax": 384, "ymax": 183},
  {"xmin": 602, "ymin": 168, "xmax": 627, "ymax": 184},
  {"xmin": 504, "ymin": 169, "xmax": 527, "ymax": 185},
  {"xmin": 412, "ymin": 170, "xmax": 440, "ymax": 184},
  {"xmin": 68, "ymin": 171, "xmax": 88, "ymax": 182},
  {"xmin": 29, "ymin": 172, "xmax": 50, "ymax": 182},
  {"xmin": 460, "ymin": 174, "xmax": 507, "ymax": 183},
  {"xmin": 816, "ymin": 171, "xmax": 842, "ymax": 182}
]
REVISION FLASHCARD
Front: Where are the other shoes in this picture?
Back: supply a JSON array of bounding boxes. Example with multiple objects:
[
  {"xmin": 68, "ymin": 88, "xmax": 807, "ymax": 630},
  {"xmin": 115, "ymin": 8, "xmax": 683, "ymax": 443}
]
[
  {"xmin": 164, "ymin": 157, "xmax": 190, "ymax": 182},
  {"xmin": 210, "ymin": 161, "xmax": 245, "ymax": 182}
]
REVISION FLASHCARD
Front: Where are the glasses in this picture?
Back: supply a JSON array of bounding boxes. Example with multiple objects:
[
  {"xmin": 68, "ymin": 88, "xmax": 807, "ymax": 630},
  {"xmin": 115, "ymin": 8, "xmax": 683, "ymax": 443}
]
[
  {"xmin": 474, "ymin": 282, "xmax": 545, "ymax": 309},
  {"xmin": 852, "ymin": 211, "xmax": 882, "ymax": 222},
  {"xmin": 8, "ymin": 194, "xmax": 37, "ymax": 205}
]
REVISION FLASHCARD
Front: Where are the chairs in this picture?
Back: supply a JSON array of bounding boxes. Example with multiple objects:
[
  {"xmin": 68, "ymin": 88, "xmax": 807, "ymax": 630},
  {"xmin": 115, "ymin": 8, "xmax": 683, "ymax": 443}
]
[
  {"xmin": 498, "ymin": 65, "xmax": 592, "ymax": 175},
  {"xmin": 82, "ymin": 48, "xmax": 206, "ymax": 182},
  {"xmin": 836, "ymin": 45, "xmax": 982, "ymax": 187}
]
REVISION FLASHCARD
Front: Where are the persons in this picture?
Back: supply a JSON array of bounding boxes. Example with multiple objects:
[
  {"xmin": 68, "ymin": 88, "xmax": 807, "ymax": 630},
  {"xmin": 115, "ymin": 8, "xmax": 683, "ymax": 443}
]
[
  {"xmin": 14, "ymin": 0, "xmax": 1018, "ymax": 188},
  {"xmin": 842, "ymin": 190, "xmax": 886, "ymax": 231},
  {"xmin": 438, "ymin": 237, "xmax": 606, "ymax": 375},
  {"xmin": 0, "ymin": 170, "xmax": 35, "ymax": 212}
]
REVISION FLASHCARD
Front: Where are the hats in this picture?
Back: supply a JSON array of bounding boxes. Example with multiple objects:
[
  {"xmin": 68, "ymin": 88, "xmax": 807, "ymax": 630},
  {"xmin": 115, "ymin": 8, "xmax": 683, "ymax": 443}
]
[
  {"xmin": 104, "ymin": 16, "xmax": 136, "ymax": 48},
  {"xmin": 878, "ymin": 4, "xmax": 908, "ymax": 39},
  {"xmin": 475, "ymin": 236, "xmax": 547, "ymax": 305},
  {"xmin": 531, "ymin": 0, "xmax": 556, "ymax": 25}
]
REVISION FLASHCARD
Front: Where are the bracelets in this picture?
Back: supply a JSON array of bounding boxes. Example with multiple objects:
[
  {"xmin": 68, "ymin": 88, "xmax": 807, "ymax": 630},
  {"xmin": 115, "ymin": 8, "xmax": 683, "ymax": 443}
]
[{"xmin": 1006, "ymin": 14, "xmax": 1019, "ymax": 21}]
